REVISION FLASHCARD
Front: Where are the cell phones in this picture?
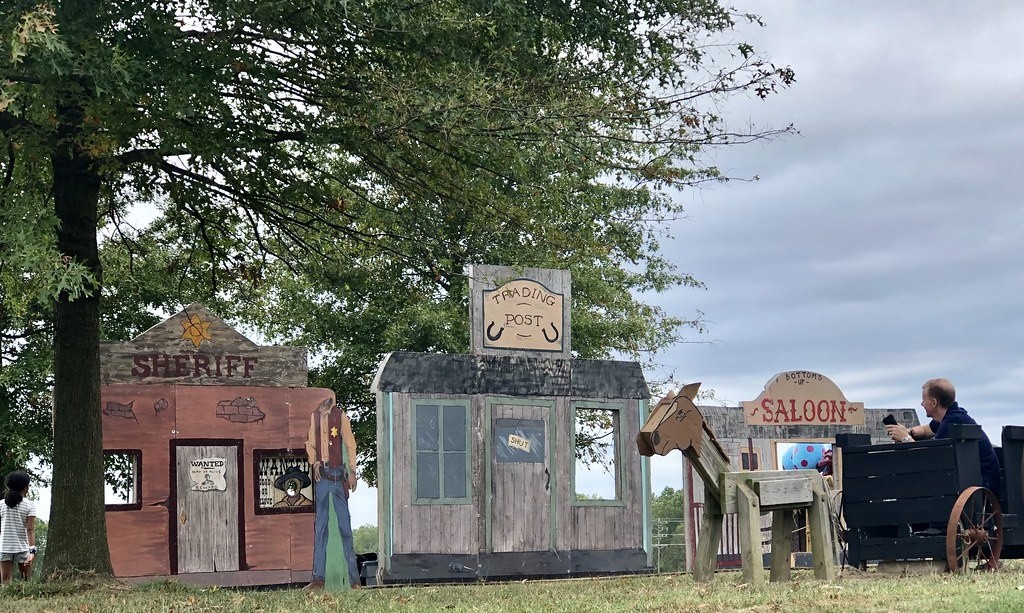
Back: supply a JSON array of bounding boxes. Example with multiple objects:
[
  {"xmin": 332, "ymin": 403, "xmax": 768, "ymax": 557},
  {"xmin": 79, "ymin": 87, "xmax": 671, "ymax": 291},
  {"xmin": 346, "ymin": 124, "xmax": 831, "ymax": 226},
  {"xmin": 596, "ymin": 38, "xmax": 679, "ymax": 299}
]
[{"xmin": 883, "ymin": 414, "xmax": 898, "ymax": 427}]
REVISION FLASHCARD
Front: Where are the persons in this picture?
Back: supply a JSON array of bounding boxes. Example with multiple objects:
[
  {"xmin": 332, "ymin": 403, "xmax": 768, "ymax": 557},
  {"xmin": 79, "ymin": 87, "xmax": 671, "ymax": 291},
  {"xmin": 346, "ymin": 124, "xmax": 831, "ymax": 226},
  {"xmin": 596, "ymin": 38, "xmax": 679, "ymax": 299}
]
[
  {"xmin": 817, "ymin": 450, "xmax": 834, "ymax": 476},
  {"xmin": 0, "ymin": 471, "xmax": 37, "ymax": 585},
  {"xmin": 885, "ymin": 378, "xmax": 1000, "ymax": 512}
]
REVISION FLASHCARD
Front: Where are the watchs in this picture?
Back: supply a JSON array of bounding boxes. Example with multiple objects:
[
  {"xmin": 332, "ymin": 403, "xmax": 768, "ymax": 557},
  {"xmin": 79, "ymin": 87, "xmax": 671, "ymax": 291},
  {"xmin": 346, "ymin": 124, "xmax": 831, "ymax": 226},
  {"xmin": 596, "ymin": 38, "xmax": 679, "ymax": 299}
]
[{"xmin": 902, "ymin": 434, "xmax": 911, "ymax": 443}]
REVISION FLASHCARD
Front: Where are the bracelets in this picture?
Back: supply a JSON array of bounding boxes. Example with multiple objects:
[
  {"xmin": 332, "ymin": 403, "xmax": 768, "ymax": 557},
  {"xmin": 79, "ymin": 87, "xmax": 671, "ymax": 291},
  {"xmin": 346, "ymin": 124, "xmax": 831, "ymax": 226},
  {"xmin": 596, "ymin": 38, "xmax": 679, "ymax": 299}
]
[{"xmin": 30, "ymin": 545, "xmax": 37, "ymax": 554}]
